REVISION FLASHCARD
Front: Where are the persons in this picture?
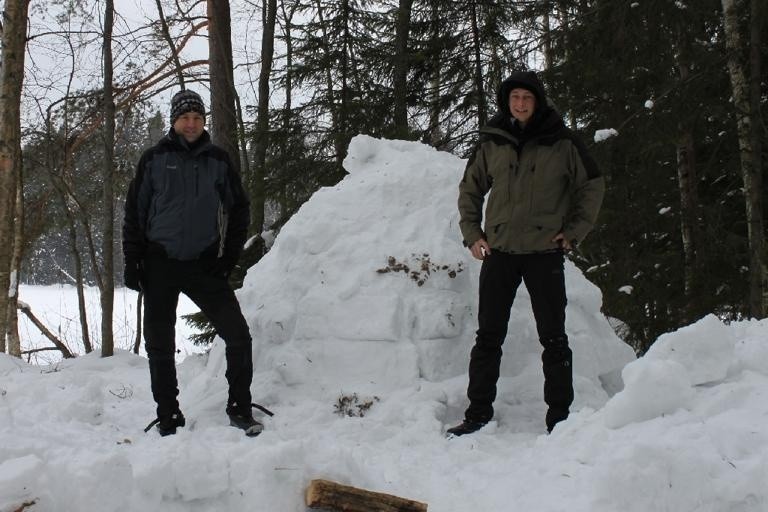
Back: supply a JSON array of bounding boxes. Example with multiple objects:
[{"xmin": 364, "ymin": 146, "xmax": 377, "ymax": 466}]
[
  {"xmin": 122, "ymin": 89, "xmax": 265, "ymax": 437},
  {"xmin": 447, "ymin": 71, "xmax": 605, "ymax": 438}
]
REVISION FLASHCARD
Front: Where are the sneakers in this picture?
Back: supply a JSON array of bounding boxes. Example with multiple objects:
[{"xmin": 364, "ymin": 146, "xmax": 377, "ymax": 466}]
[
  {"xmin": 157, "ymin": 409, "xmax": 186, "ymax": 436},
  {"xmin": 447, "ymin": 419, "xmax": 484, "ymax": 436},
  {"xmin": 229, "ymin": 414, "xmax": 263, "ymax": 435}
]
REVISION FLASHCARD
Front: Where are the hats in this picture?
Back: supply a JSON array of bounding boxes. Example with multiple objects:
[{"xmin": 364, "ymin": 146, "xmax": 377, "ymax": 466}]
[{"xmin": 170, "ymin": 89, "xmax": 206, "ymax": 128}]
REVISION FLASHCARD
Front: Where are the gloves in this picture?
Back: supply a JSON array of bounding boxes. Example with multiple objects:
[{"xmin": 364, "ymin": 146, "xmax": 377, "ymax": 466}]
[
  {"xmin": 124, "ymin": 259, "xmax": 142, "ymax": 292},
  {"xmin": 210, "ymin": 257, "xmax": 234, "ymax": 280}
]
